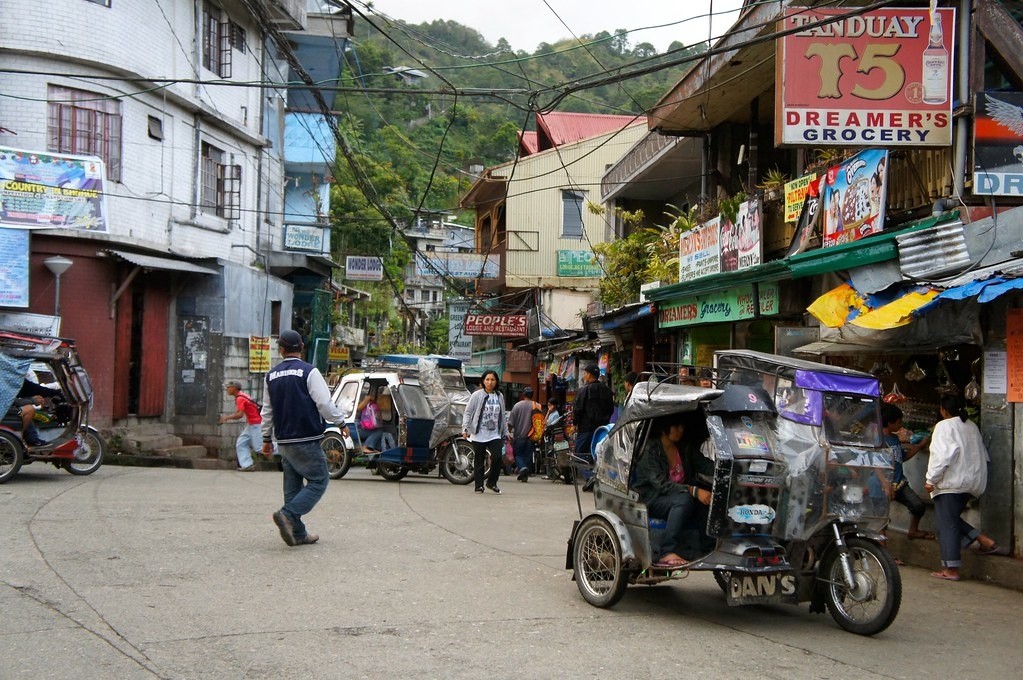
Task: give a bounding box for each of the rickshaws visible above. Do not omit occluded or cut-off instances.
[319,355,492,485]
[566,350,902,637]
[0,327,106,485]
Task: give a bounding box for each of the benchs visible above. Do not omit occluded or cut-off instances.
[593,484,698,530]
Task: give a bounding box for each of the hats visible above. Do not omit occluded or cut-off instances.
[585,365,600,376]
[523,387,533,394]
[226,381,242,389]
[622,372,640,382]
[276,330,302,348]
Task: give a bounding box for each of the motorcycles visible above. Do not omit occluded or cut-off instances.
[543,410,574,485]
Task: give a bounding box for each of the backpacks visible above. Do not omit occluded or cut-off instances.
[527,401,545,441]
[360,400,384,430]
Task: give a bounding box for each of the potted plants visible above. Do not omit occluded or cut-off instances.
[761,162,788,199]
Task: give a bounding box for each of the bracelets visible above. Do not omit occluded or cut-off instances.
[692,486,698,498]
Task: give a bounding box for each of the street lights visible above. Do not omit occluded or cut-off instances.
[43,256,73,317]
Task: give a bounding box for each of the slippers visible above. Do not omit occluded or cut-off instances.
[654,553,689,567]
[974,546,1000,555]
[908,530,935,540]
[930,571,961,581]
[894,559,906,565]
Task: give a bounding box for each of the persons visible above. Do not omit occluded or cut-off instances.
[355,394,383,453]
[869,403,936,565]
[507,386,543,482]
[219,379,279,472]
[462,370,512,494]
[632,412,717,566]
[572,365,615,493]
[541,398,560,480]
[14,378,57,447]
[736,207,759,250]
[624,371,641,391]
[827,188,843,234]
[260,329,350,546]
[925,393,1000,580]
[680,365,712,387]
[869,157,885,219]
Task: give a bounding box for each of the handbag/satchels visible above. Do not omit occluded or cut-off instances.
[869,361,981,405]
[503,441,514,465]
[258,405,262,413]
[895,427,930,444]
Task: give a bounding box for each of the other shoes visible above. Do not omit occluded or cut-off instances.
[475,486,484,493]
[28,438,53,448]
[582,476,595,491]
[541,475,549,480]
[517,469,528,483]
[273,510,297,546]
[486,483,501,494]
[296,531,319,544]
[237,464,255,472]
[362,445,380,453]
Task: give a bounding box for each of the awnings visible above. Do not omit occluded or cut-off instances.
[101,248,220,306]
